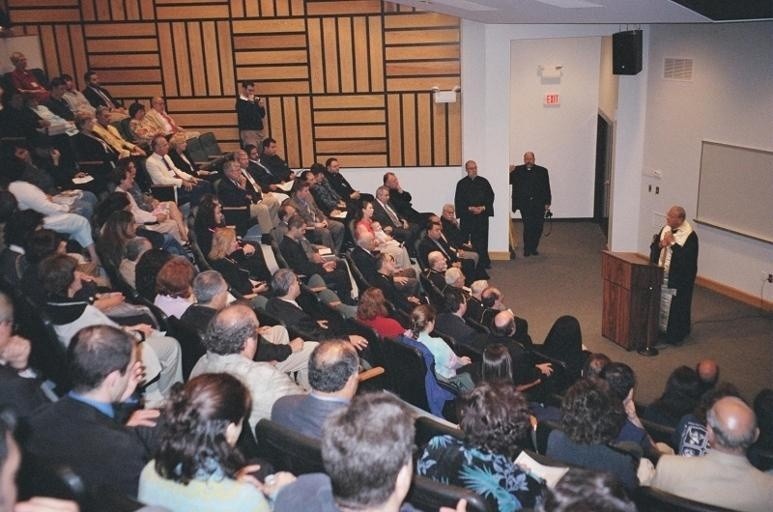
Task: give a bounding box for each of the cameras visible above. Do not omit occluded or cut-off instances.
[254,96,260,101]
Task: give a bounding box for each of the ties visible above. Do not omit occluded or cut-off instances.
[161,156,183,180]
[98,88,115,108]
[160,112,178,130]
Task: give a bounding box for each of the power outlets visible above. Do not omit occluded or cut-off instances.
[768,274,773,283]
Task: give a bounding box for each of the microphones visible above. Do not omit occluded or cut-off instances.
[650,234,659,248]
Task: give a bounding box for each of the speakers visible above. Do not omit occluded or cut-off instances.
[612,30,642,75]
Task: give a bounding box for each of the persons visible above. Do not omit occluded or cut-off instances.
[0,50,772,511]
